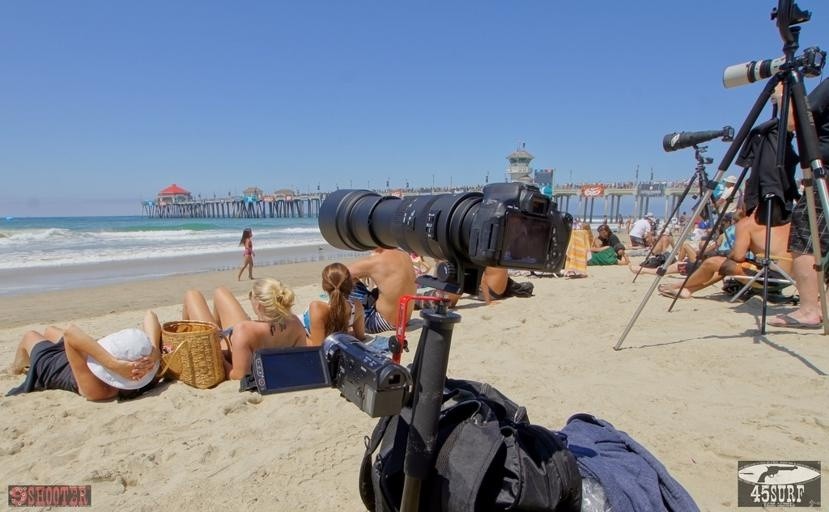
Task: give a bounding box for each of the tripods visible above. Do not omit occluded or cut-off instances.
[632,164,732,284]
[613,69,829,351]
[666,162,796,335]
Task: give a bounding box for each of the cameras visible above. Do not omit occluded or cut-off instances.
[239,331,413,418]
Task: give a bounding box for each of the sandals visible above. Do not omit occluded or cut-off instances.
[565,270,588,278]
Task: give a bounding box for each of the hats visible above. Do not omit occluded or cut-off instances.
[86,328,159,391]
[725,176,738,185]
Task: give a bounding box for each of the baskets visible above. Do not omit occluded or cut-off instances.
[157,320,234,389]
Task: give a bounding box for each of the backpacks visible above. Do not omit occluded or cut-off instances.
[359,372,587,511]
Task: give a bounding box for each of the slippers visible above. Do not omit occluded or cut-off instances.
[766,314,821,330]
[658,283,674,291]
[662,289,681,300]
